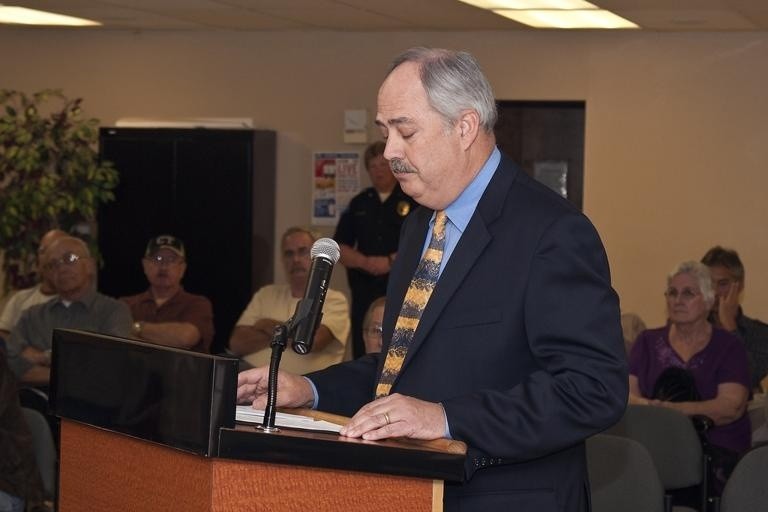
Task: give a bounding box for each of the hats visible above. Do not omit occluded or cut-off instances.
[144,233,185,258]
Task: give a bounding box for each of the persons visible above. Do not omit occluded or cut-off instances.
[362,294,386,354]
[700,245,768,404]
[236,46,631,511]
[228,225,351,376]
[334,140,419,360]
[1,338,46,512]
[626,259,752,511]
[8,234,132,387]
[0,228,71,342]
[117,235,214,350]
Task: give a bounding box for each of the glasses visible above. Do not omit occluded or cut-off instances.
[149,254,176,264]
[363,323,383,339]
[42,252,87,275]
[36,246,46,255]
[664,287,696,302]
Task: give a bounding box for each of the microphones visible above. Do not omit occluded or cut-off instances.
[292,237,344,355]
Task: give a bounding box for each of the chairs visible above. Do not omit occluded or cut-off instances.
[585,433,673,512]
[605,404,708,512]
[714,447,768,512]
[21,406,59,512]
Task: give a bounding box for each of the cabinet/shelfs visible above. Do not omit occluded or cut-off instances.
[97,126,277,353]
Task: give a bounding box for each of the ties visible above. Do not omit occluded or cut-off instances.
[375,211,447,398]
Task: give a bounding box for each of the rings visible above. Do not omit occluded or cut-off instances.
[383,412,391,425]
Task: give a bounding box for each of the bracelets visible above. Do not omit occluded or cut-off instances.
[43,345,53,366]
[133,319,143,338]
